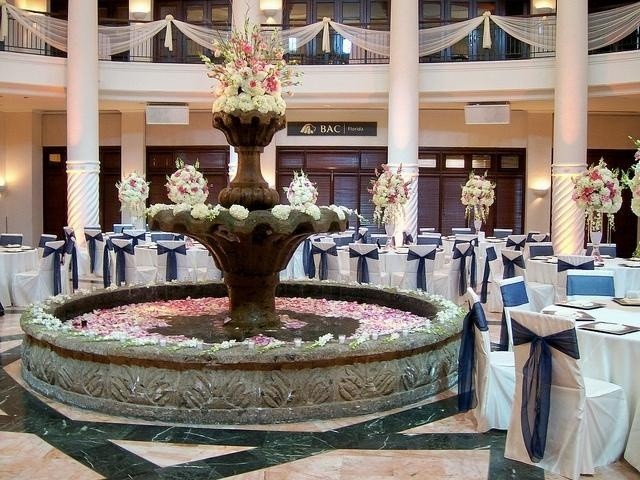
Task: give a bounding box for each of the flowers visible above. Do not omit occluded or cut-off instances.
[622,136,640,221]
[284,173,318,210]
[368,162,412,224]
[147,203,355,223]
[462,171,498,222]
[200,1,301,114]
[570,156,622,222]
[117,174,152,214]
[168,159,209,207]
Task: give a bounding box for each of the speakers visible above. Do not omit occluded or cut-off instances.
[145,106,189,124]
[464,104,510,124]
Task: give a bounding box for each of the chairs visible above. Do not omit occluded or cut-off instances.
[0,223,639,479]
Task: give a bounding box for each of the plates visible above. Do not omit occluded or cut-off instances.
[598,255,614,259]
[577,321,640,337]
[555,300,607,310]
[531,255,560,264]
[613,297,639,306]
[5,244,36,253]
[595,262,604,267]
[618,257,640,268]
[542,310,595,322]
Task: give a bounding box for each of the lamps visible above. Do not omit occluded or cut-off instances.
[133,12,147,19]
[263,8,277,19]
[536,8,554,14]
